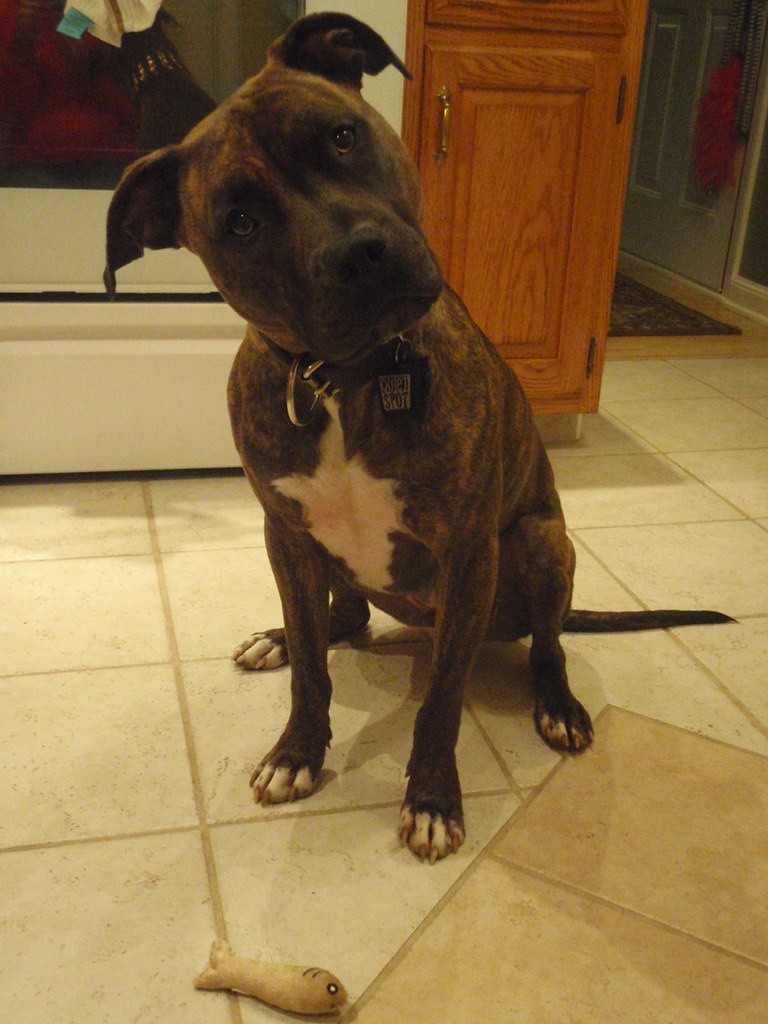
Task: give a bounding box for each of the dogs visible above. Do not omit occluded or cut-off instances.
[103,10,740,863]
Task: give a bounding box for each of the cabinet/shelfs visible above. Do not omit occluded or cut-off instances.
[403,1,646,444]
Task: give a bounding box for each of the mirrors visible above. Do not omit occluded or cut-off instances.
[0,0,306,194]
